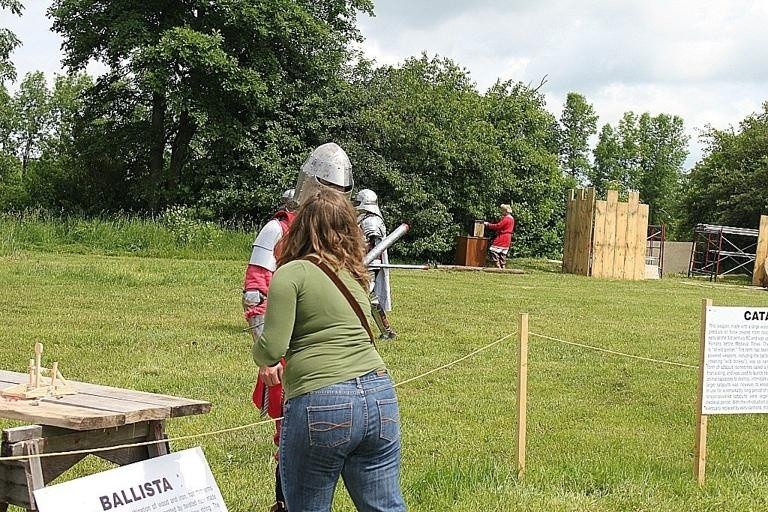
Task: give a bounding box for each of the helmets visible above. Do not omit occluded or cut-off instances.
[284,142,354,206]
[354,189,385,221]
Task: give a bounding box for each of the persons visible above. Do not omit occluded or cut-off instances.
[484,204,514,269]
[350,187,397,342]
[243,142,354,512]
[252,189,407,512]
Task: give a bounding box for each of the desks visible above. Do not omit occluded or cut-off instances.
[456,234,492,266]
[0,365,213,510]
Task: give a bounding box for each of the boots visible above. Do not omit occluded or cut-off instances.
[372,298,400,342]
